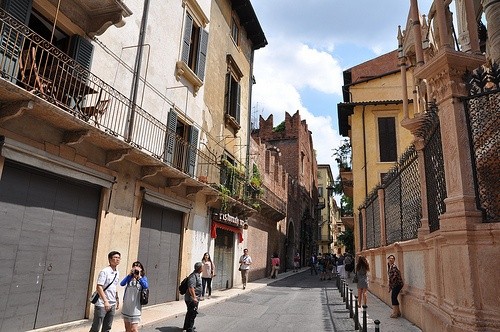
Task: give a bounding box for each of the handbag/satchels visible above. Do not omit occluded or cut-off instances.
[239,268,241,271]
[90,291,99,304]
[141,289,149,305]
[275,266,280,269]
[352,271,357,283]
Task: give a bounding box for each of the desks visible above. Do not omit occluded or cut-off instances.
[39,63,99,114]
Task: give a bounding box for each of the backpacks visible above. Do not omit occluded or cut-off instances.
[179,277,188,295]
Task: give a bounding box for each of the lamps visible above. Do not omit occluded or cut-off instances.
[243,224,248,230]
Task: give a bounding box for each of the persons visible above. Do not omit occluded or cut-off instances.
[270,252,280,279]
[356,256,370,308]
[239,249,252,289]
[387,255,403,318]
[309,252,339,281]
[120,261,148,332]
[183,261,204,332]
[200,252,215,300]
[90,251,121,332]
[293,253,300,272]
[338,252,354,278]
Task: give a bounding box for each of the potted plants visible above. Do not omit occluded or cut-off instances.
[338,163,351,172]
[250,176,261,190]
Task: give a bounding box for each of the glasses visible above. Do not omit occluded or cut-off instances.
[135,263,140,266]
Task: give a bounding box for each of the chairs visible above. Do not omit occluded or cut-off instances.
[81,99,111,129]
[18,47,57,106]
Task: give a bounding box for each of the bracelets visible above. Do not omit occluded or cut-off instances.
[116,297,118,299]
